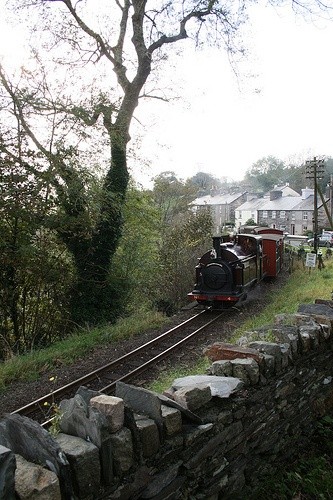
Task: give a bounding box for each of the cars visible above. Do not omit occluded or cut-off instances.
[306,234,333,247]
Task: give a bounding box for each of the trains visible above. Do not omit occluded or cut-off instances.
[187,224,284,315]
